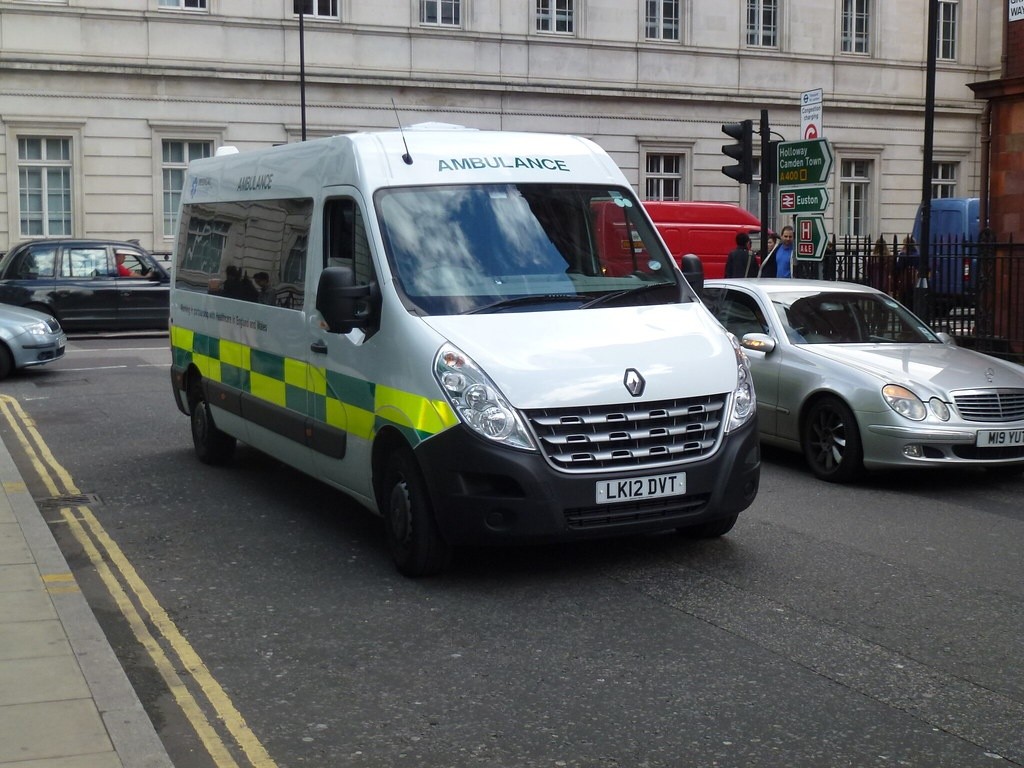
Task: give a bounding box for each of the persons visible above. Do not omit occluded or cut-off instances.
[866,237,920,331]
[116,254,152,278]
[768,226,835,281]
[723,233,760,279]
[208,266,282,308]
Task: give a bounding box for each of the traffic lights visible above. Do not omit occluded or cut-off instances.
[720,117,754,183]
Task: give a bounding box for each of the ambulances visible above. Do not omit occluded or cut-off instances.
[168,121,765,581]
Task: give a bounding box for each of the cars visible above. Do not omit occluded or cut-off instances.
[0,236,181,331]
[696,277,1024,486]
[0,300,68,382]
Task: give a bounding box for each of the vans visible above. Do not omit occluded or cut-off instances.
[899,197,989,315]
[583,200,785,284]
